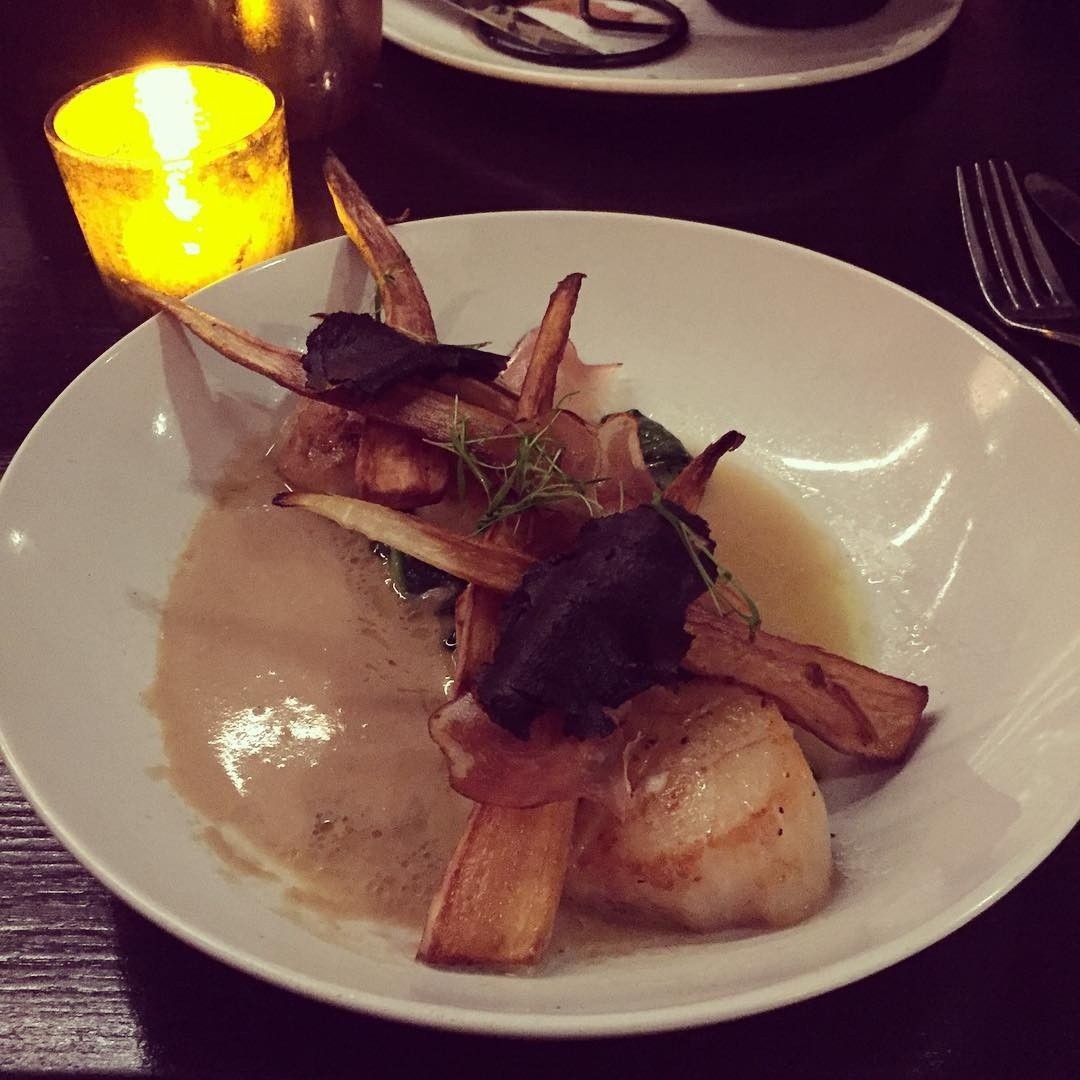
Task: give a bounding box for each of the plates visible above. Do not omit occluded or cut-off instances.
[337,0,963,94]
[1,208,1079,1038]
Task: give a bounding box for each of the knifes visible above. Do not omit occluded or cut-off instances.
[1024,171,1079,245]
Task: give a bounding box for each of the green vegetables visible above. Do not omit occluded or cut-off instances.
[420,394,762,633]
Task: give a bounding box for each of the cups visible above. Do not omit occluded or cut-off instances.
[44,60,294,322]
[152,1,384,145]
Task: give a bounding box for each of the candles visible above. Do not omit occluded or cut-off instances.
[54,58,289,305]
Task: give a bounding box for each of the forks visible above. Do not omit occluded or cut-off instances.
[957,161,1080,355]
